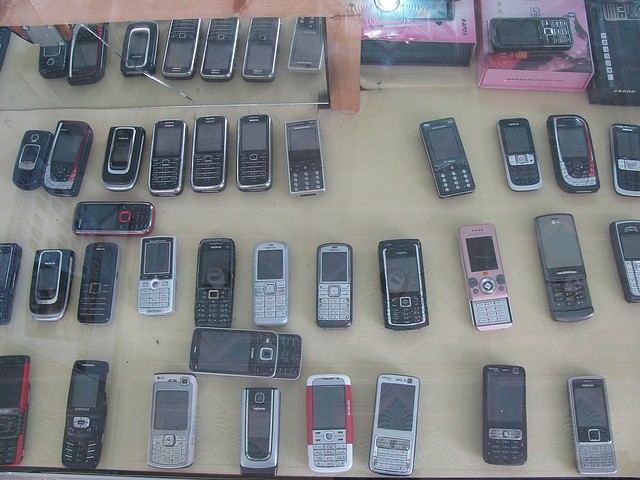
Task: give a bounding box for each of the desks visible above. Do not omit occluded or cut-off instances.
[0,0,368,114]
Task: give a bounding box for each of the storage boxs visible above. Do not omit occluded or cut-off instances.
[582,2,639,106]
[476,2,596,93]
[358,0,478,69]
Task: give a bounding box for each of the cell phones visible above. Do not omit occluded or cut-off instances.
[609,122,640,199]
[148,119,188,197]
[566,374,619,476]
[497,118,544,192]
[200,16,240,82]
[188,328,302,379]
[367,373,421,474]
[137,236,176,317]
[420,117,478,197]
[43,118,93,197]
[287,17,325,75]
[67,24,108,87]
[608,217,640,303]
[457,225,514,330]
[77,242,121,325]
[378,238,431,331]
[235,114,274,192]
[120,23,159,79]
[239,384,280,477]
[194,236,235,329]
[0,241,23,326]
[147,371,197,475]
[243,17,281,82]
[37,30,70,79]
[11,128,50,193]
[534,214,596,323]
[0,351,31,469]
[252,241,288,328]
[192,116,228,193]
[284,116,326,199]
[305,373,354,475]
[59,358,110,469]
[481,361,529,468]
[546,112,600,193]
[488,15,573,51]
[71,204,154,237]
[315,243,354,329]
[102,126,145,191]
[29,246,75,320]
[160,18,202,82]
